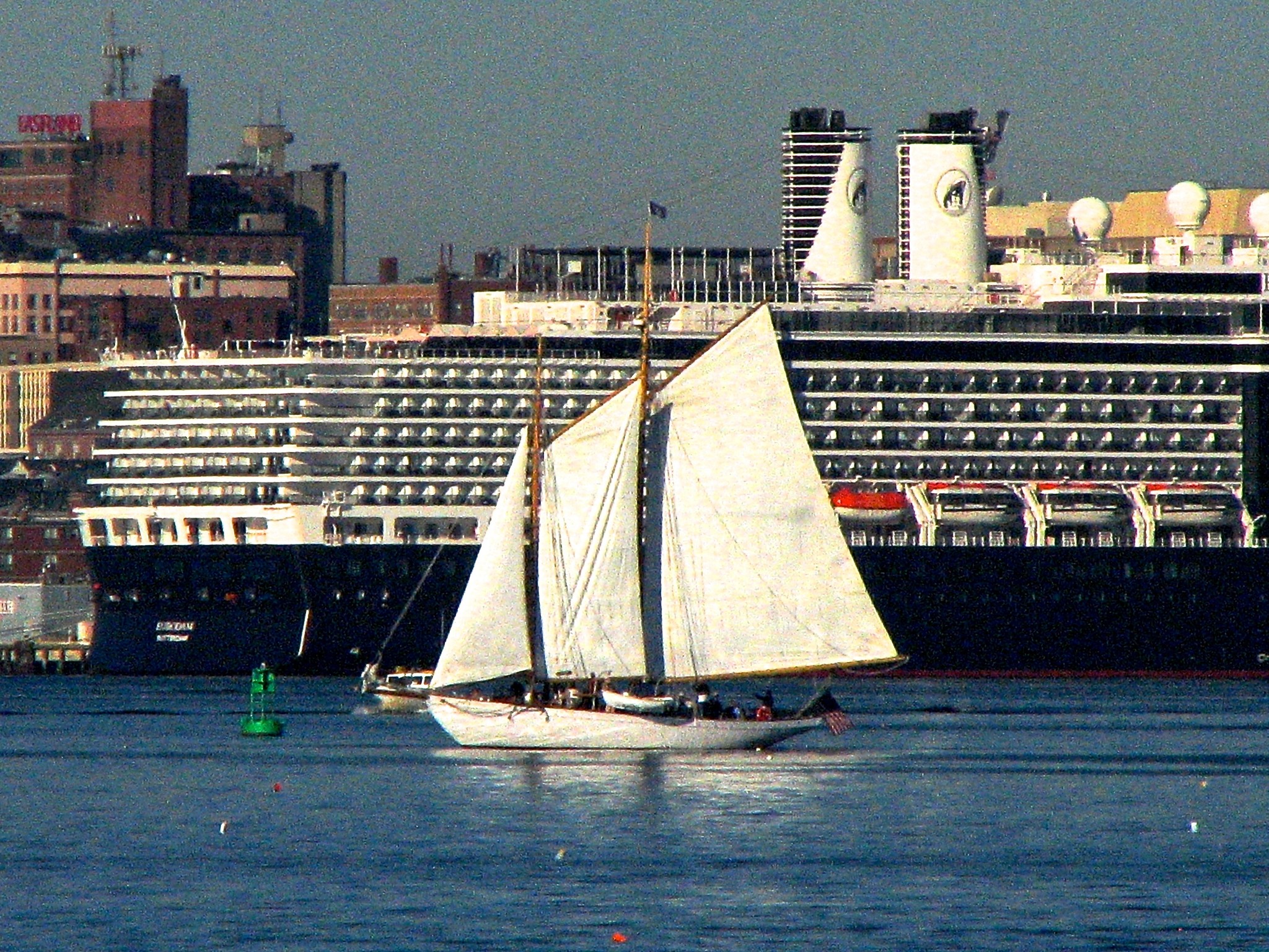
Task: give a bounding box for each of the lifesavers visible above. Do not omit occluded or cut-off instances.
[756,706,772,721]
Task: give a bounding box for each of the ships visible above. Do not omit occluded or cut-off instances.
[76,296,1268,681]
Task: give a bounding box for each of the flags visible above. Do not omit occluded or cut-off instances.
[649,201,667,219]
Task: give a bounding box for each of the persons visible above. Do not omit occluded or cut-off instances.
[754,689,773,710]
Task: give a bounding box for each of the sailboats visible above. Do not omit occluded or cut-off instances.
[422,206,905,750]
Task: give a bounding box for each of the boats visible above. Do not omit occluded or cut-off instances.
[1035,478,1129,527]
[834,487,908,524]
[923,483,1024,529]
[369,671,435,712]
[1143,483,1243,530]
